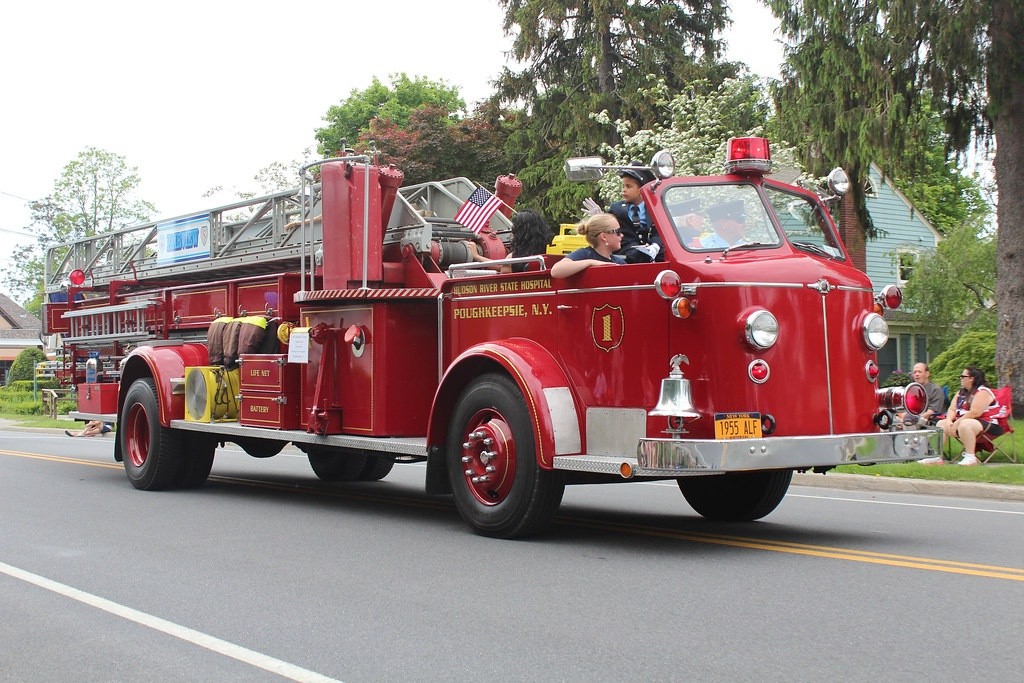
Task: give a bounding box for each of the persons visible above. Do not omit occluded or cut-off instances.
[467,161,751,279]
[65,420,114,437]
[897,363,1002,466]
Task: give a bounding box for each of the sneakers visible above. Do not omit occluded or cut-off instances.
[917,455,943,465]
[957,452,977,465]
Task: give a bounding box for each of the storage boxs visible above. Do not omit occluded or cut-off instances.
[77,383,119,414]
[234,352,302,430]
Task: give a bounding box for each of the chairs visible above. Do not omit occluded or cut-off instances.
[927,385,951,462]
[949,385,1017,463]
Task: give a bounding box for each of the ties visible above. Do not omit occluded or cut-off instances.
[631,206,641,229]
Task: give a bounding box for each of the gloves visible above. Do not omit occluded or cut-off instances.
[580,196,602,215]
[647,242,661,260]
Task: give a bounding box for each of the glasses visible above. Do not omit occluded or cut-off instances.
[959,375,970,379]
[594,227,622,237]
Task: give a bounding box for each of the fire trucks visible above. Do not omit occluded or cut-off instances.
[33,138,945,543]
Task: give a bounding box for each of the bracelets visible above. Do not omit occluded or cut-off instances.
[955,421,959,425]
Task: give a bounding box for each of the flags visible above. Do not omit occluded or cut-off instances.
[454,186,503,235]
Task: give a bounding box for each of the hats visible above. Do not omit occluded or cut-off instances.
[616,160,656,186]
[668,197,705,217]
[706,199,748,221]
